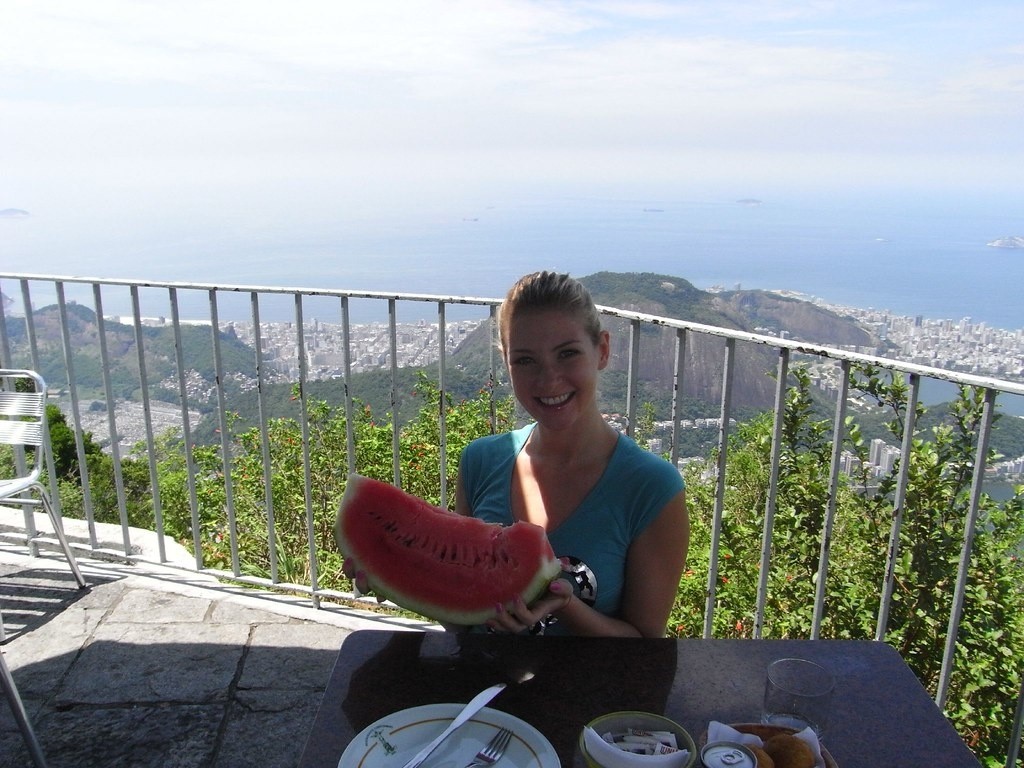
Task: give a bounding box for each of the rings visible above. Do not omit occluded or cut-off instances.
[528,614,560,636]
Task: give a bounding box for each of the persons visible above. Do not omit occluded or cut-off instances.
[342,270,691,639]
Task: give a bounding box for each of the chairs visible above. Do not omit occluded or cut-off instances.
[0,367,87,587]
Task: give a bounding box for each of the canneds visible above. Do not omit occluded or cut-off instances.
[697,740,758,768]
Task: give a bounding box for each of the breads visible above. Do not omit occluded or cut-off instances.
[742,743,775,768]
[763,733,817,768]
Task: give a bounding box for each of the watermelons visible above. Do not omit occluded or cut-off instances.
[330,472,561,626]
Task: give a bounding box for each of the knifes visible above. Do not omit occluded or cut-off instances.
[403,683,506,768]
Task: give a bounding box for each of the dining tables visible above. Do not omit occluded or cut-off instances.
[297,629,985,767]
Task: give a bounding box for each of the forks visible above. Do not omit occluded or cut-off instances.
[464,727,514,768]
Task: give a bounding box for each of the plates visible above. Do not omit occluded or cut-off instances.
[337,703,562,768]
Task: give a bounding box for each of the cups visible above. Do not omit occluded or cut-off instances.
[760,658,834,743]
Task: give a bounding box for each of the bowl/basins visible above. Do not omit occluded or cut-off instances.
[579,711,696,768]
[699,723,837,768]
